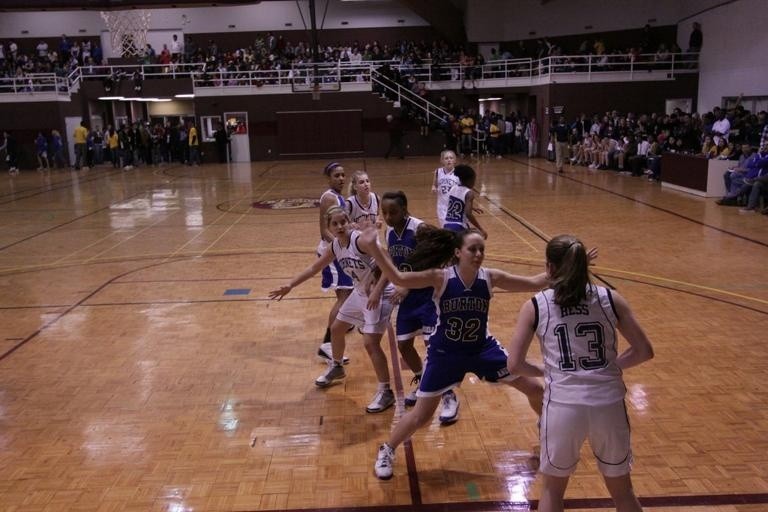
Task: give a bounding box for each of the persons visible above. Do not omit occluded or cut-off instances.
[507,235,654,512]
[432,151,458,228]
[356,214,598,478]
[381,190,461,423]
[345,170,386,231]
[1,22,768,215]
[444,165,489,241]
[317,161,354,366]
[267,205,396,413]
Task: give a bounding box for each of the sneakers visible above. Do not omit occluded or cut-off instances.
[366,389,395,413]
[316,342,350,363]
[374,445,394,479]
[439,394,461,422]
[315,364,348,387]
[405,374,422,406]
